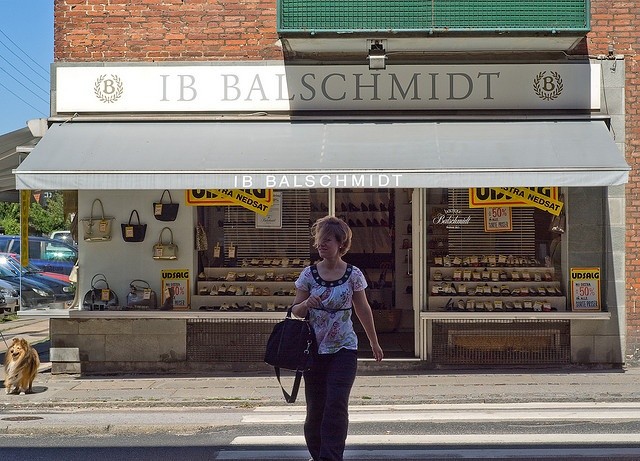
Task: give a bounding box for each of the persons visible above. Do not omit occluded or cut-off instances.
[289,216,384,461]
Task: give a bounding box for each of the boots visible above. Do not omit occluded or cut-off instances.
[226,245,237,267]
[211,246,224,267]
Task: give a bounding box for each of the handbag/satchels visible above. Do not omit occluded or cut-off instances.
[82,274,119,310]
[69,258,79,283]
[549,211,565,235]
[263,303,318,403]
[153,190,179,221]
[79,199,116,242]
[152,227,178,261]
[121,209,147,242]
[127,280,157,311]
[372,300,402,334]
[70,212,78,245]
[195,221,208,251]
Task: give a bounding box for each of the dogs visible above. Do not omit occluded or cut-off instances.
[3,337,40,395]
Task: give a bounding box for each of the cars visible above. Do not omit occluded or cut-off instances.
[51,231,78,247]
[0,254,76,301]
[0,252,73,283]
[0,280,20,315]
[0,254,56,308]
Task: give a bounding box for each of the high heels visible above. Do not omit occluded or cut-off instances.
[200,284,296,296]
[436,298,557,312]
[338,216,387,226]
[434,255,540,267]
[198,272,299,282]
[335,202,386,211]
[431,282,563,296]
[433,268,552,281]
[241,258,311,268]
[199,302,293,312]
[311,203,328,212]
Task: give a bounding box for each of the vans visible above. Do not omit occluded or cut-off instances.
[0,236,78,262]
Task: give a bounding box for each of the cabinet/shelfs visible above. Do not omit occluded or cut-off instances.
[190,260,309,312]
[399,201,447,295]
[427,255,568,318]
[351,263,401,334]
[202,190,393,259]
